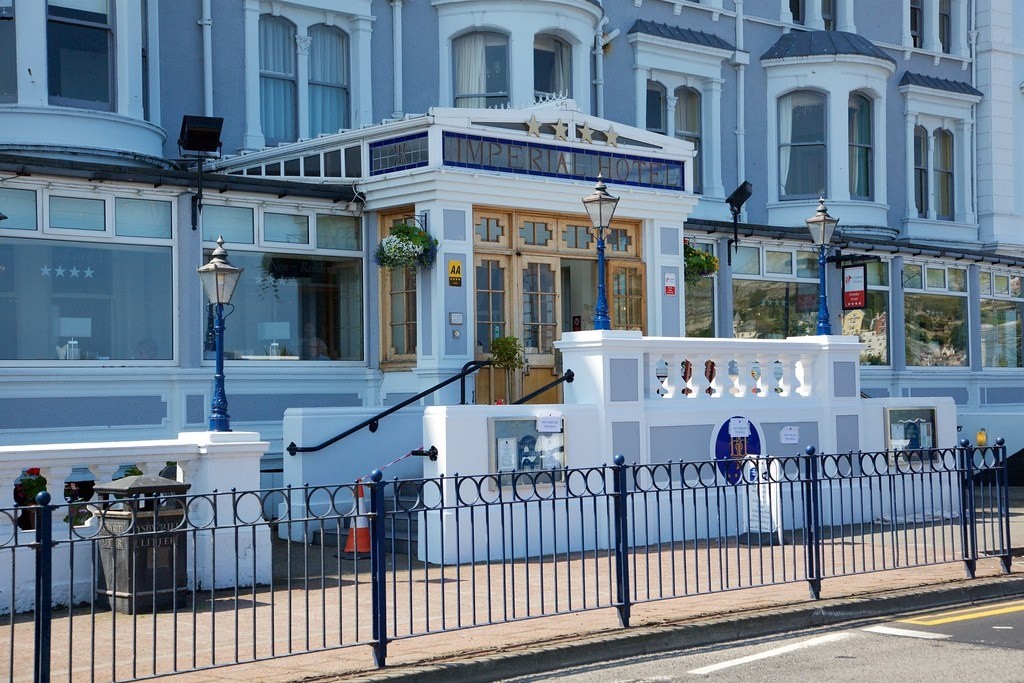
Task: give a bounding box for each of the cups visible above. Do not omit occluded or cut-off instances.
[67,341,79,359]
[270,342,280,361]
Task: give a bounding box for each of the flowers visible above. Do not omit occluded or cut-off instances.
[684,237,720,292]
[370,223,439,286]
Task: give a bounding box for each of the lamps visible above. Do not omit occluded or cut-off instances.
[177,116,225,232]
[725,181,753,267]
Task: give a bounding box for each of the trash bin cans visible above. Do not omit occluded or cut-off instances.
[93,474,193,615]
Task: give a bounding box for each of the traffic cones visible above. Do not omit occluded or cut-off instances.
[333,477,374,560]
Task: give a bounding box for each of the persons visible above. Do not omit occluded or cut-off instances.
[137,339,158,360]
[297,321,331,360]
